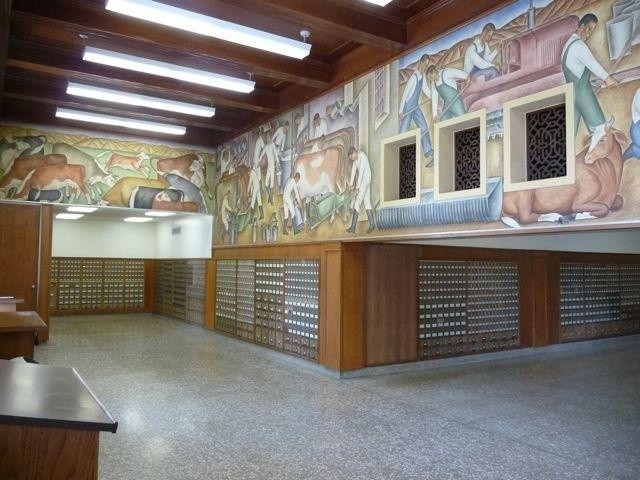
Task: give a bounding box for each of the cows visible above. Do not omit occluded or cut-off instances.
[1,132,215,215]
[288,124,357,229]
[498,112,628,228]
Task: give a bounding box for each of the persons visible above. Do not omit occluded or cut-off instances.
[560,13,621,137]
[218,113,377,243]
[622,86,640,164]
[397,23,500,167]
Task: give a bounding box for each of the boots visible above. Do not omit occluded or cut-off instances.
[365,209,375,233]
[346,209,359,233]
[283,216,301,236]
[247,204,264,224]
[424,150,434,167]
[265,185,276,206]
[221,230,228,243]
